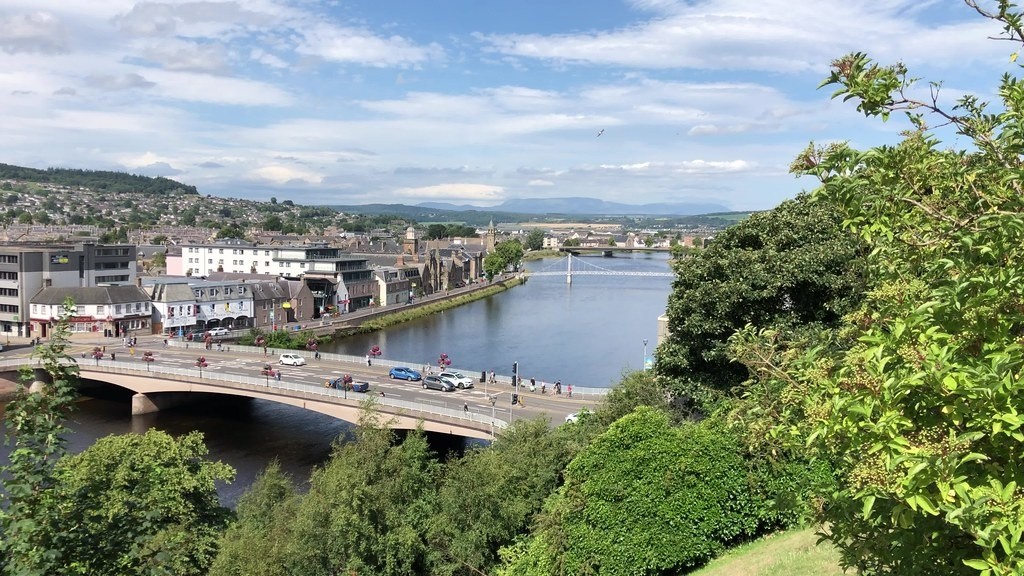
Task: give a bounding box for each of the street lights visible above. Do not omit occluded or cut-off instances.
[643,339,649,373]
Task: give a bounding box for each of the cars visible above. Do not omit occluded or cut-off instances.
[389,366,422,382]
[279,353,306,367]
[207,327,231,336]
[565,411,596,424]
[439,371,475,390]
[422,375,456,392]
[184,328,210,342]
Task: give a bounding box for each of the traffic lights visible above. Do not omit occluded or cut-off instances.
[512,362,517,373]
[512,394,518,405]
[511,375,517,386]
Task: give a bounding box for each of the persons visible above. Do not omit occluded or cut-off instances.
[122,334,136,347]
[277,371,281,380]
[488,370,495,385]
[542,381,545,393]
[216,341,222,351]
[568,384,572,397]
[464,402,468,411]
[163,339,168,348]
[264,346,267,354]
[315,349,318,359]
[552,380,561,395]
[531,377,535,391]
